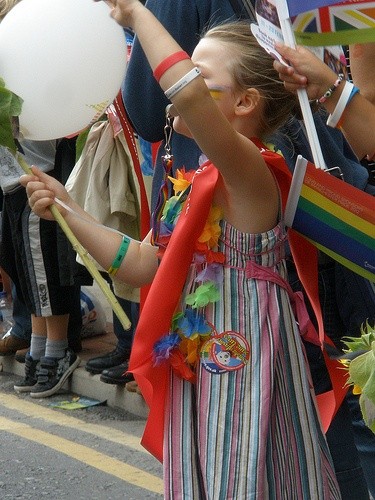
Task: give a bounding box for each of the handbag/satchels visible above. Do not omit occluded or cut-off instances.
[79,286,107,339]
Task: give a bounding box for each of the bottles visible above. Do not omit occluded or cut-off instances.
[0,292,15,336]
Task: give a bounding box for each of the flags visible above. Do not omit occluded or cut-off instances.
[285,0,375,46]
[283,155,375,283]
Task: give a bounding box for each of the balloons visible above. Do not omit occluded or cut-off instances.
[0,0,127,141]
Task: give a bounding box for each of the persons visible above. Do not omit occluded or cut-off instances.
[124,0,375,500]
[0,0,141,399]
[17,0,341,500]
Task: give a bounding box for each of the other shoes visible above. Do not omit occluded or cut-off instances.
[0,336,30,356]
[16,349,29,363]
[100,359,135,384]
[86,349,131,374]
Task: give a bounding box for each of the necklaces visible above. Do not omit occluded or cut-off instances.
[153,141,285,383]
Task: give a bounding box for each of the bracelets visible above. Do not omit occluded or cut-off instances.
[316,73,344,105]
[108,235,130,276]
[152,50,190,84]
[326,80,360,128]
[163,67,201,99]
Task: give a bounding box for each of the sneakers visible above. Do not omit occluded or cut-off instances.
[14,351,40,391]
[29,348,81,397]
